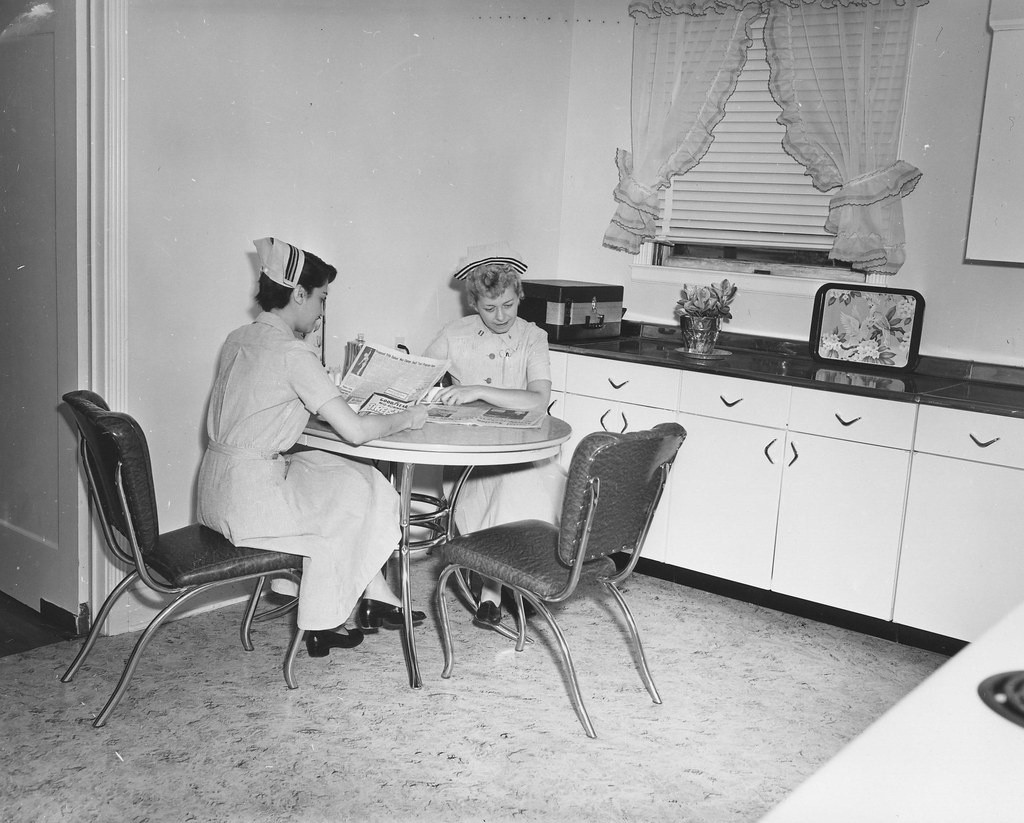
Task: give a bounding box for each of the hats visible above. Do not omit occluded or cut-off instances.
[453,241,528,281]
[253,237,305,289]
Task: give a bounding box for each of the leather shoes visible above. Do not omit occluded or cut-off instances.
[506,587,536,618]
[476,583,501,625]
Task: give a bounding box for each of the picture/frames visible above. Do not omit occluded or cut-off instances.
[808,282,925,376]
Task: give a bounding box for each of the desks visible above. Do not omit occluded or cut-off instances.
[295,400,573,691]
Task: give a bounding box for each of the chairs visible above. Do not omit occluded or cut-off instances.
[59,390,305,728]
[435,422,687,738]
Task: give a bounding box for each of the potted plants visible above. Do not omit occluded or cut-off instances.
[673,278,737,354]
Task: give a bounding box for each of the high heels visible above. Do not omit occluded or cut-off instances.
[307,629,365,658]
[360,595,427,629]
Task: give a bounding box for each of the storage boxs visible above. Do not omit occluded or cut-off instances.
[517,278,625,343]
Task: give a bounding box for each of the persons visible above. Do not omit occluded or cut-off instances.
[195,237,428,658]
[419,256,572,628]
[357,349,372,374]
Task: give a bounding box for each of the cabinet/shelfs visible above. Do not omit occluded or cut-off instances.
[541,351,1024,658]
